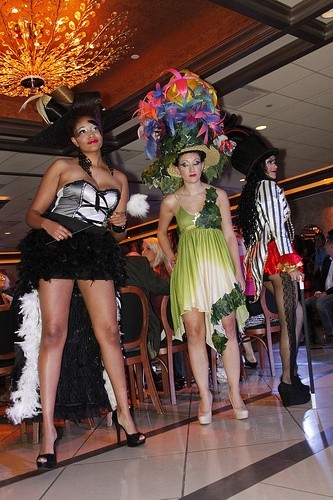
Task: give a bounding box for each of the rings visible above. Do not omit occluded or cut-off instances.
[58,235,62,238]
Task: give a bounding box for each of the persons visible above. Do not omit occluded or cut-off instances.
[239,154,310,405]
[121,237,194,396]
[295,229,333,341]
[235,232,279,367]
[10,114,147,468]
[0,275,13,304]
[157,148,249,424]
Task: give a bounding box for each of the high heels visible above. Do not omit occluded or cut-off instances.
[244,355,258,368]
[227,391,248,419]
[278,381,311,407]
[198,390,213,424]
[36,425,63,470]
[279,374,310,392]
[112,408,146,447]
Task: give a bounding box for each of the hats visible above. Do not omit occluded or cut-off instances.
[229,124,280,180]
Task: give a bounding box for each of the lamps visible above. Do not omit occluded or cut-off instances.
[0,0,138,96]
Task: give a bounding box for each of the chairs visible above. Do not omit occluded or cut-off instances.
[0,286,281,445]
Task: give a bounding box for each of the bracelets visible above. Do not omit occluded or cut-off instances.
[111,223,127,233]
